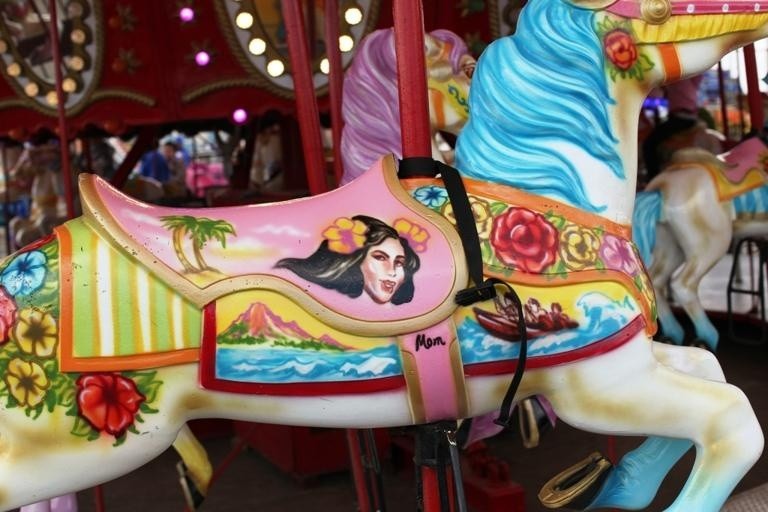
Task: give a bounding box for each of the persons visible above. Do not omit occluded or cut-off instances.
[660,71,705,136]
[140,136,192,202]
